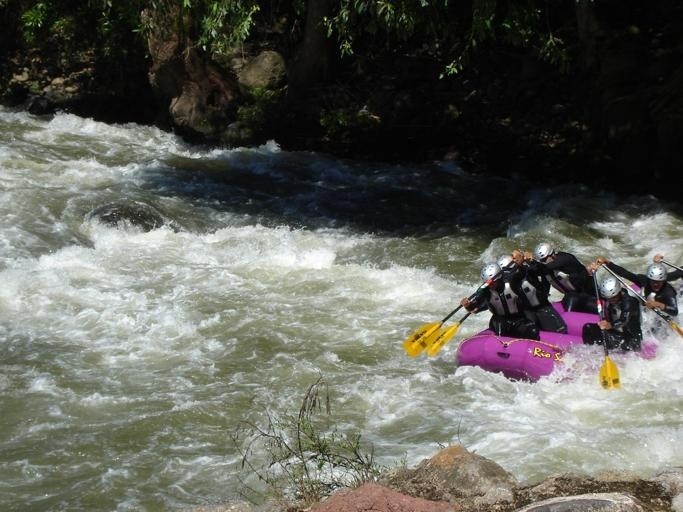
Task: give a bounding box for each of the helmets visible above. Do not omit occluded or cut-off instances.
[646,263,667,281]
[534,242,553,260]
[599,277,622,299]
[480,255,516,282]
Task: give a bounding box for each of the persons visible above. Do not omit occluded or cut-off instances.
[460,243,683,355]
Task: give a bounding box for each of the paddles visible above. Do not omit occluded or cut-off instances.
[591,267,620,392]
[426,291,487,359]
[598,258,683,339]
[401,254,514,358]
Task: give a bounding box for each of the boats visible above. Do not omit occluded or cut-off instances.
[456,283,680,383]
